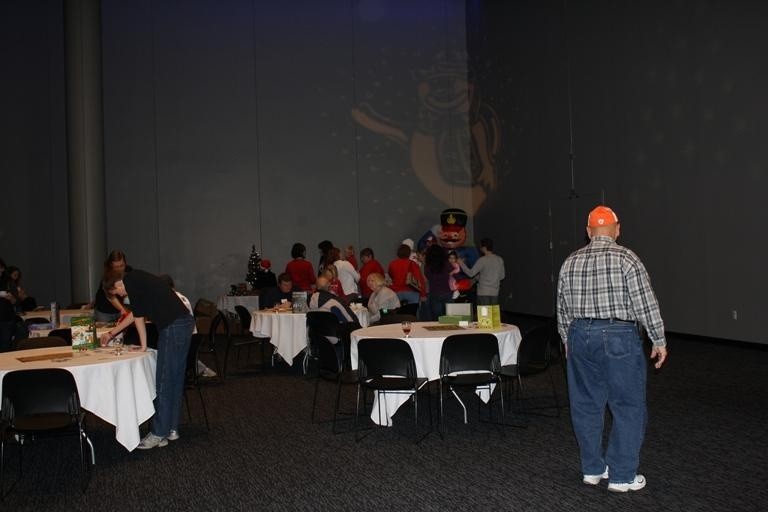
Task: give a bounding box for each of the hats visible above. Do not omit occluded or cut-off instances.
[588,205,618,228]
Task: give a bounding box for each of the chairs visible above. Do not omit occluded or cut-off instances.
[24,316,52,328]
[440,332,501,442]
[0,369,89,467]
[47,328,73,346]
[306,310,361,436]
[233,304,252,335]
[354,337,433,444]
[216,309,268,373]
[500,323,562,430]
[184,332,235,433]
[190,299,220,367]
[122,321,159,352]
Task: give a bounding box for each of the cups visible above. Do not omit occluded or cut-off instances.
[273,303,280,315]
[401,321,412,339]
[112,338,123,356]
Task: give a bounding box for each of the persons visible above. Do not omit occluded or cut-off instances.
[424,244,455,322]
[327,247,361,295]
[307,277,362,330]
[367,273,401,324]
[286,243,316,307]
[388,244,427,317]
[359,248,385,298]
[0,258,6,278]
[94,249,133,322]
[267,272,301,308]
[342,244,358,270]
[0,266,25,314]
[103,269,196,450]
[317,240,333,277]
[256,259,277,287]
[415,247,431,321]
[556,206,668,494]
[457,238,505,321]
[160,275,198,377]
[447,252,460,300]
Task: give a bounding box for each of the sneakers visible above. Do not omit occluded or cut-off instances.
[166,429,179,441]
[583,465,609,485]
[137,432,169,449]
[606,475,646,493]
